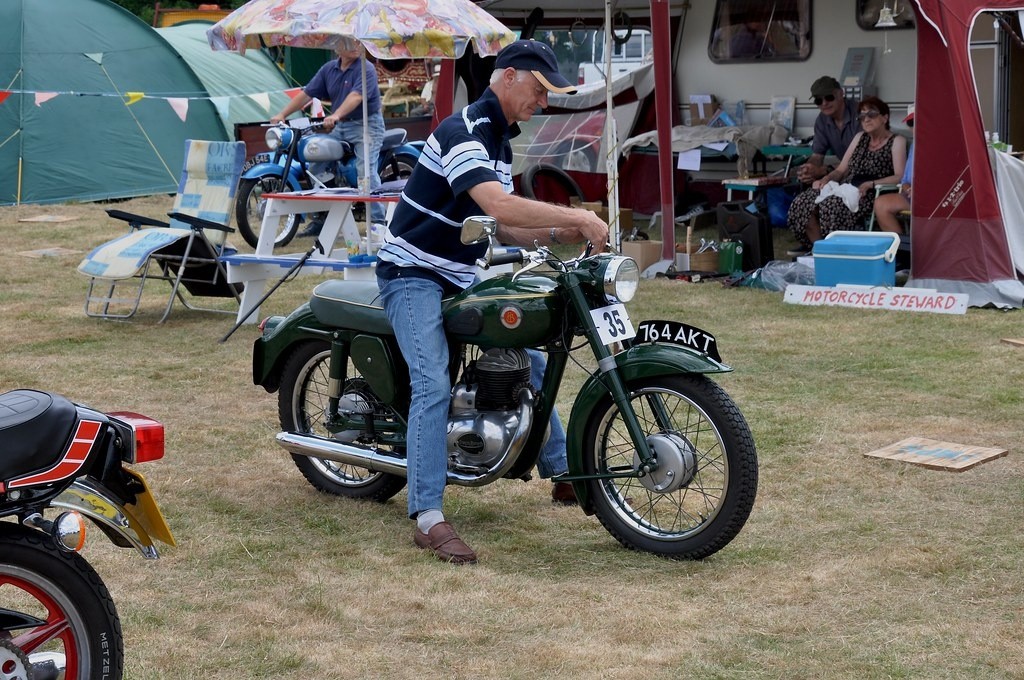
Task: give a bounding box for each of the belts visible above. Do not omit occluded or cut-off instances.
[339,108,381,122]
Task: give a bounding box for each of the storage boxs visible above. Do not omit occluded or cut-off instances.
[580,202,719,274]
[690,102,720,126]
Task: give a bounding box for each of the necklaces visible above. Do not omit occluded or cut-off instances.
[866,134,889,149]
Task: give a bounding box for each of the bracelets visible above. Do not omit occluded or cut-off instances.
[872,180,875,189]
[549,226,562,246]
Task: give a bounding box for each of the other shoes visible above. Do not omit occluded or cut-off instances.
[787,245,811,256]
[895,269,910,283]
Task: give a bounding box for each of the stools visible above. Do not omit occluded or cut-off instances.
[722,174,789,203]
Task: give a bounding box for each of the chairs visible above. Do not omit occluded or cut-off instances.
[867,184,910,248]
[77,139,248,326]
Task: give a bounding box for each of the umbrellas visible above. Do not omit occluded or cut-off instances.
[204,0,518,255]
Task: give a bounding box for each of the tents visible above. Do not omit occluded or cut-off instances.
[0,0,304,206]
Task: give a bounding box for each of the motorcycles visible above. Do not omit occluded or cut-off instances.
[251,215,760,562]
[0,387,176,680]
[234,121,427,250]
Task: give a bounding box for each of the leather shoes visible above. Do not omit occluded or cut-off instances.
[551,483,634,506]
[413,521,476,564]
[296,222,324,237]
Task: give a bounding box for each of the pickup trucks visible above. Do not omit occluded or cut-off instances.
[576,29,654,85]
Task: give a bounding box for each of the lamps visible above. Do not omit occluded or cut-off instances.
[875,0,897,27]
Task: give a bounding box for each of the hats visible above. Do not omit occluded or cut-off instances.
[495,38,578,95]
[902,104,914,124]
[432,64,441,77]
[809,76,839,101]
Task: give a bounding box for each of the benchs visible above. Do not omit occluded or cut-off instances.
[218,254,380,272]
[486,245,526,255]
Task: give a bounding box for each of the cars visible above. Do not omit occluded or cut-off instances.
[525,110,607,172]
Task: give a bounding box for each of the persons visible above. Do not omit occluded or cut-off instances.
[786,74,915,276]
[374,38,608,566]
[272,37,387,237]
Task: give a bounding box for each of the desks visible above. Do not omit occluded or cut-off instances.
[761,146,833,171]
[632,144,765,226]
[236,191,400,323]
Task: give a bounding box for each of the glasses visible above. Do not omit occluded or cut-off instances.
[814,93,836,106]
[906,119,914,127]
[856,110,880,121]
